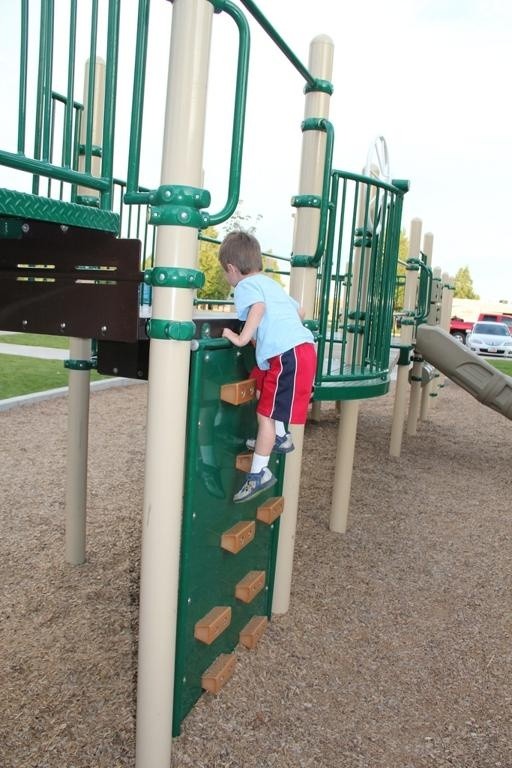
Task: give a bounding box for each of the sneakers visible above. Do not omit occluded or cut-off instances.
[233,466,277,504]
[245,431,293,454]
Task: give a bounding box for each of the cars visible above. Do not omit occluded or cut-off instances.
[465,321,512,357]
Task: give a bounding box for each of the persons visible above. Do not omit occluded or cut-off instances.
[217,229,318,504]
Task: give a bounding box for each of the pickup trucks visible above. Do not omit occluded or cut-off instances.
[451,311,512,345]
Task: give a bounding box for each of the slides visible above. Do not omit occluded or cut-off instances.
[416,323,511,420]
